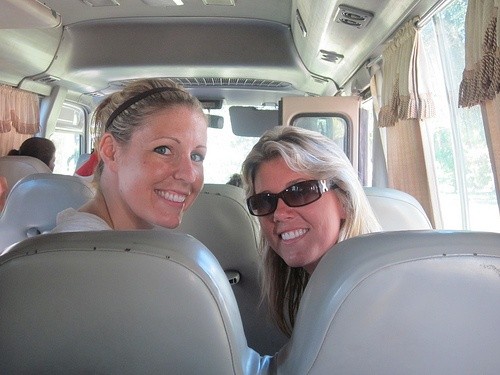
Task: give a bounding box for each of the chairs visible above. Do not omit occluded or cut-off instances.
[0,155,500,375]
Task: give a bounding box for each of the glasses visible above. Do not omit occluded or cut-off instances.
[247,179,339,217]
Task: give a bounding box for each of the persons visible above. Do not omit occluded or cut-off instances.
[241,125,382,341]
[7,137,56,172]
[0,80,207,255]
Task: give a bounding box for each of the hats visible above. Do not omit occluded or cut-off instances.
[75,151,97,177]
